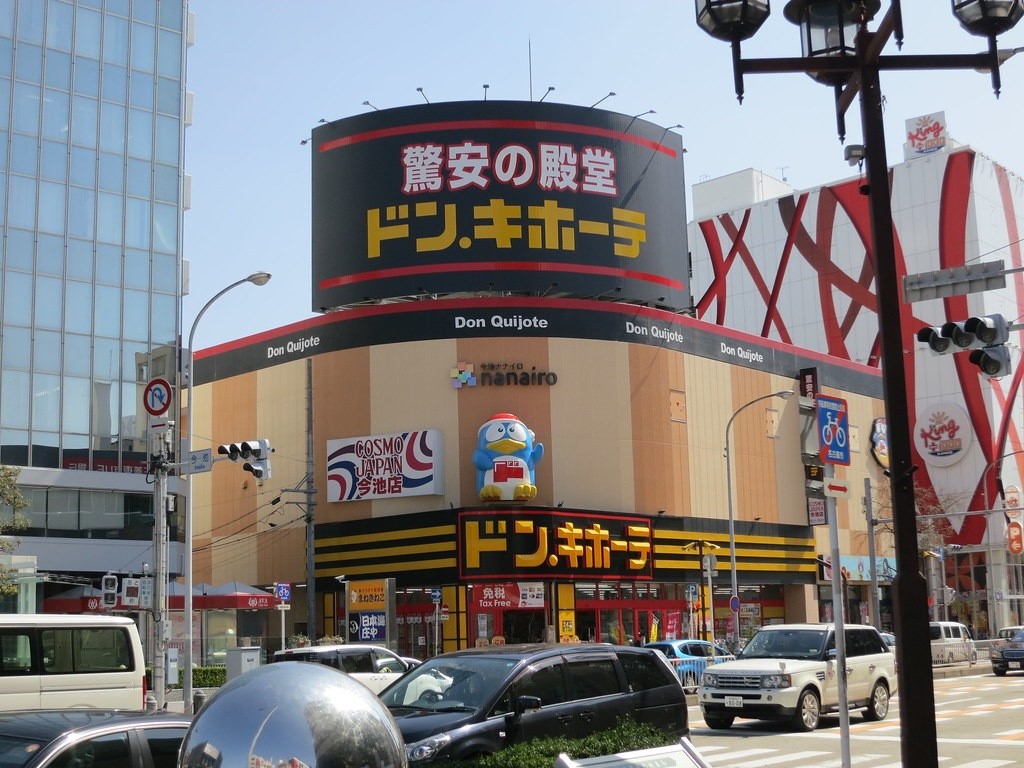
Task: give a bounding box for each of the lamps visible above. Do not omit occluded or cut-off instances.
[540,86,556,102]
[681,148,689,153]
[319,119,332,125]
[633,110,658,120]
[642,296,666,304]
[417,87,430,104]
[482,84,490,103]
[299,138,313,145]
[592,91,616,108]
[320,307,329,311]
[362,101,380,112]
[487,282,495,297]
[594,286,623,300]
[666,124,684,131]
[676,305,697,312]
[417,286,438,300]
[363,296,373,301]
[539,282,560,298]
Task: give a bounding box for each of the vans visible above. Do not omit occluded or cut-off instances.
[376,645,692,768]
[0,614,148,712]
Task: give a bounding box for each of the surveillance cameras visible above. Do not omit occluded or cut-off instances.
[335,575,345,580]
[704,542,720,550]
[682,542,698,552]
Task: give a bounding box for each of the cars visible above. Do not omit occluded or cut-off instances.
[373,657,454,695]
[643,640,736,694]
[929,621,977,664]
[274,645,444,704]
[990,628,1024,676]
[995,626,1024,649]
[0,708,194,768]
[880,633,898,672]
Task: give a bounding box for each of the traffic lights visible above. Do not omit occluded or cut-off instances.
[242,463,272,481]
[968,346,1012,378]
[946,544,964,552]
[218,439,270,462]
[917,313,1009,357]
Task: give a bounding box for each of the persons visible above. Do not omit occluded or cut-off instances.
[634,633,645,647]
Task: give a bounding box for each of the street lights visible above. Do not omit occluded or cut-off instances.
[183,273,273,717]
[698,0,1024,768]
[725,390,796,650]
[682,540,721,642]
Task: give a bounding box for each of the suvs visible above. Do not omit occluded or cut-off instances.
[696,622,899,731]
